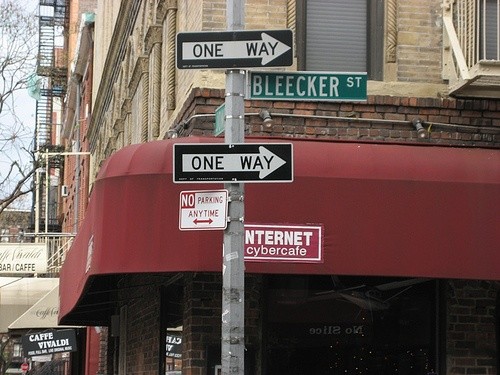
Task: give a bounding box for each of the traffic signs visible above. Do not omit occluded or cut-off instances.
[179,189,229,231]
[174,143,294,183]
[215,104,225,136]
[176,30,294,70]
[244,71,369,101]
